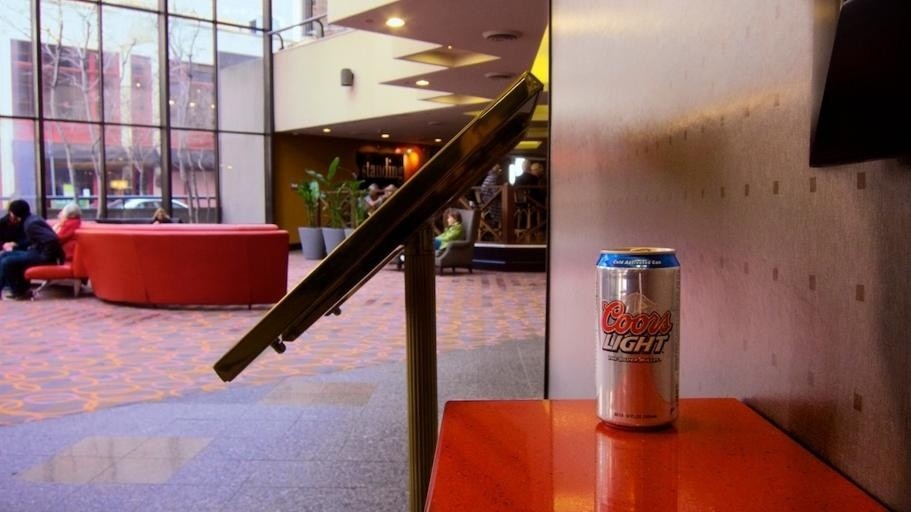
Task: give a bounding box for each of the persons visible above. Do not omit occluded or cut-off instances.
[150,208,172,224]
[365,184,397,217]
[0,200,65,300]
[481,164,503,228]
[435,212,464,251]
[514,163,545,208]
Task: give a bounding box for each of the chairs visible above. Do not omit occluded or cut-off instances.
[435,208,480,276]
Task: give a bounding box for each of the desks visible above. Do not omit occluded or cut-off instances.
[423,397,892,512]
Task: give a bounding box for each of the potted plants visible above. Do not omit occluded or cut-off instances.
[291,155,369,260]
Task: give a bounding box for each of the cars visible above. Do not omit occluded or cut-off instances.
[106,197,191,210]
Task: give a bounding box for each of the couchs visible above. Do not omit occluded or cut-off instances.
[82,221,290,305]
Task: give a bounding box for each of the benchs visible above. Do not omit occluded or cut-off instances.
[24,218,82,300]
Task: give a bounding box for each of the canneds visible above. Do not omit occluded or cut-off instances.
[594,246,682,428]
